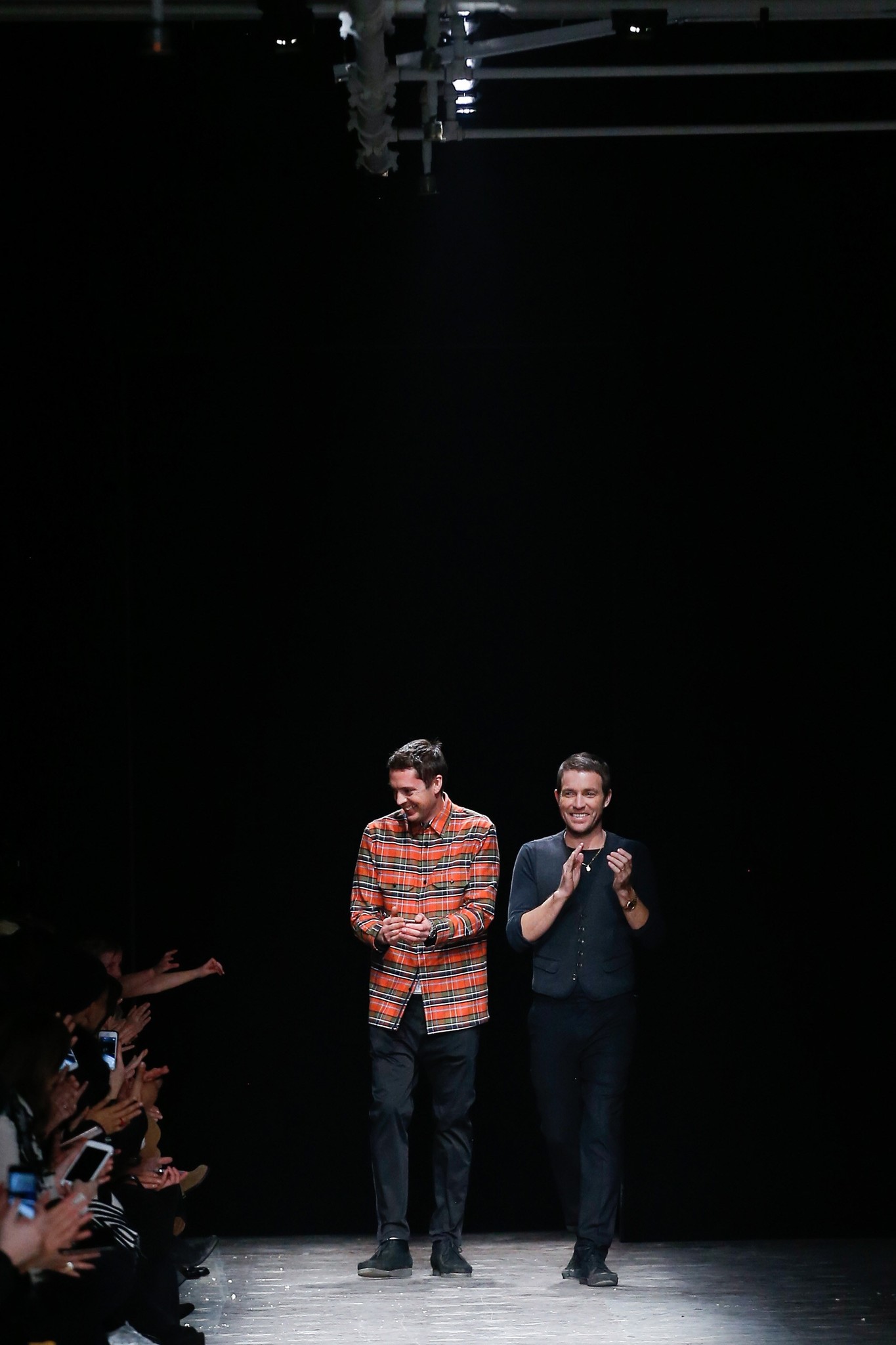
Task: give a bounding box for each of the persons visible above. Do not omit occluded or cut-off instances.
[349,739,501,1278]
[506,751,659,1286]
[0,935,224,1345]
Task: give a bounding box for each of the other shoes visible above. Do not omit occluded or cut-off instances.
[579,1246,618,1286]
[160,1324,205,1345]
[358,1239,413,1277]
[178,1302,195,1320]
[168,1234,219,1268]
[431,1236,472,1277]
[560,1250,582,1279]
[178,1265,210,1279]
[179,1164,208,1195]
[563,1222,578,1238]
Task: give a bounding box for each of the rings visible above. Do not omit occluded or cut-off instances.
[119,1118,126,1127]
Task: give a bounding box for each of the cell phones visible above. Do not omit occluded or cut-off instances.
[6,1163,42,1220]
[59,1049,80,1075]
[97,1030,119,1071]
[152,1168,185,1175]
[62,1125,103,1147]
[60,1140,115,1188]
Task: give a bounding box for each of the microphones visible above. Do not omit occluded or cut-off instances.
[425,842,428,847]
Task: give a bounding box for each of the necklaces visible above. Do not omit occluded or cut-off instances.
[564,831,603,871]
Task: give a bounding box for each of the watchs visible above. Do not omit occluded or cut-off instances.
[429,920,437,940]
[623,889,637,910]
